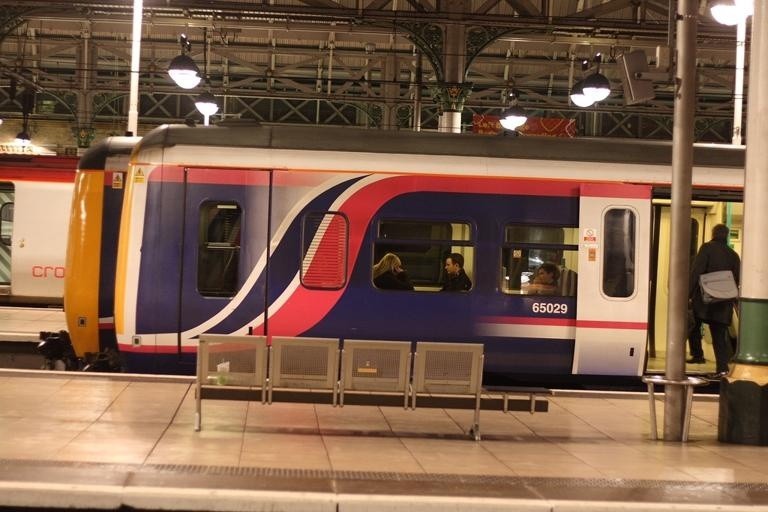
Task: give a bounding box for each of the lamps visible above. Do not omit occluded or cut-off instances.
[497,84,528,131]
[569,52,613,108]
[167,34,220,118]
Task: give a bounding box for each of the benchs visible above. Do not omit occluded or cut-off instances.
[192,332,487,443]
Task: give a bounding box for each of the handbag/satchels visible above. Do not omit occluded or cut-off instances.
[698,270,739,305]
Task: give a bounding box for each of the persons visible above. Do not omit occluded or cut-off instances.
[523,263,561,294]
[439,252,472,294]
[372,252,410,290]
[689,224,740,378]
[685,296,707,364]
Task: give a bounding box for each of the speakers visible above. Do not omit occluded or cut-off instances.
[615,49,655,107]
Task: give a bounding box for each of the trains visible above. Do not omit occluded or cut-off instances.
[0,121,767,391]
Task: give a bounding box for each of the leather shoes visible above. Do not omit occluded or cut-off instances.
[711,370,728,378]
[687,356,704,364]
